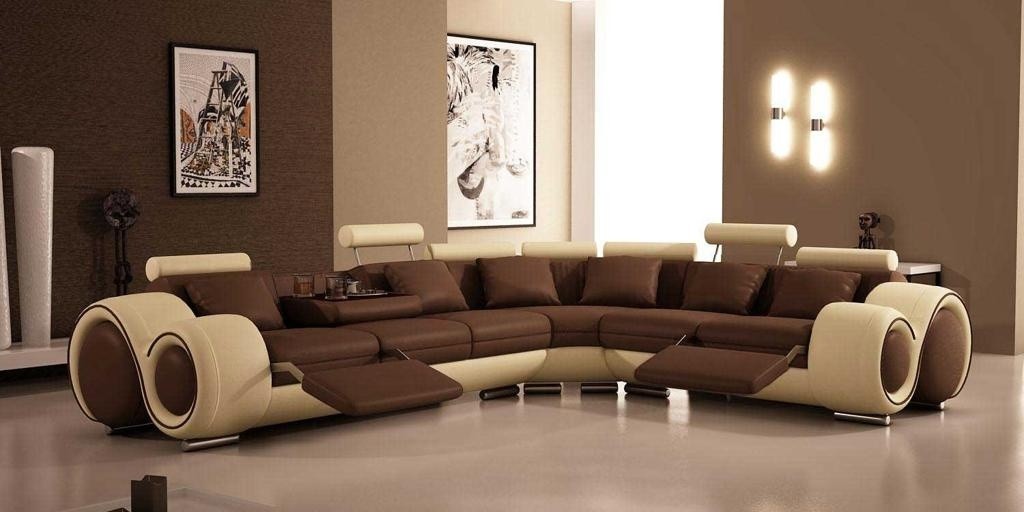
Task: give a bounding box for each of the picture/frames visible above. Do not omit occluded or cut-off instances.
[444,32,539,230]
[167,42,260,199]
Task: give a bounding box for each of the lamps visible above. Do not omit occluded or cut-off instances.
[809,78,839,174]
[771,67,795,161]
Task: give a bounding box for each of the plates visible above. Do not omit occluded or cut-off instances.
[346,290,388,297]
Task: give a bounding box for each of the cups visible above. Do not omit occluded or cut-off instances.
[292,273,315,297]
[323,277,348,300]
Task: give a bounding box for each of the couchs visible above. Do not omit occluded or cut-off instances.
[67,220,974,450]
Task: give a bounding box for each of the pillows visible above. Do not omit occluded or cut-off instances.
[338,223,424,246]
[797,245,899,274]
[476,256,563,307]
[705,223,799,250]
[145,252,254,283]
[424,242,516,261]
[579,256,662,310]
[281,292,423,326]
[768,269,860,318]
[682,261,768,314]
[523,240,600,260]
[184,274,286,332]
[604,240,696,261]
